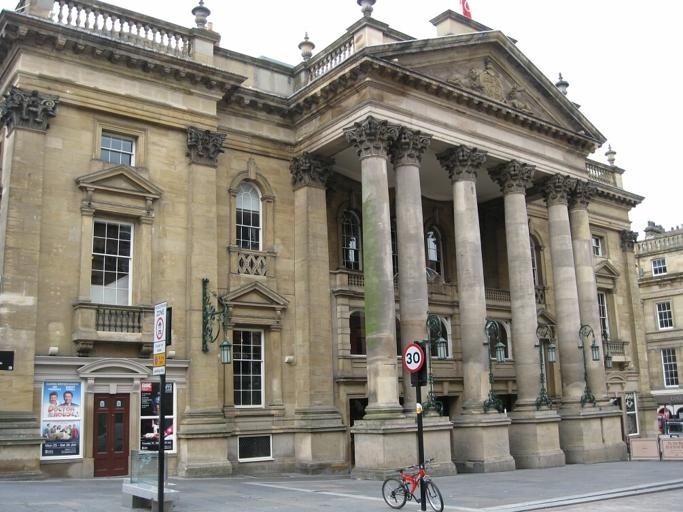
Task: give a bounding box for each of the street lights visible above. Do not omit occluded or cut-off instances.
[535,325,557,412]
[423,313,448,416]
[602,329,617,406]
[577,324,601,409]
[483,319,506,414]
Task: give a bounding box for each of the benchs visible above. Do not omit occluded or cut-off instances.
[122,483,180,512]
[123,478,176,507]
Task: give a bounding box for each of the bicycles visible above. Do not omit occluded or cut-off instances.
[382,457,445,512]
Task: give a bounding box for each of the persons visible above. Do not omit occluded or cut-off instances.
[45,391,60,416]
[43,422,78,442]
[53,390,79,408]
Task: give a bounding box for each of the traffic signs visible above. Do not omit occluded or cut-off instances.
[402,343,424,373]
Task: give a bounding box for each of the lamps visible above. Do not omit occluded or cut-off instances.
[603,330,613,369]
[202,278,232,364]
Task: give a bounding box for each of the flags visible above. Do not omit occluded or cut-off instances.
[461,0,472,18]
